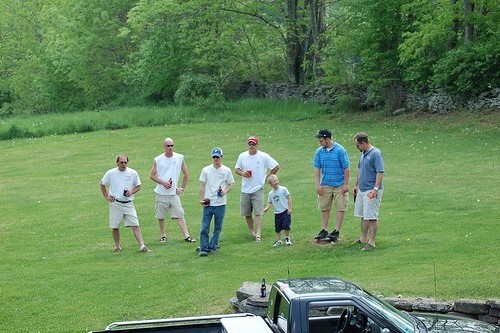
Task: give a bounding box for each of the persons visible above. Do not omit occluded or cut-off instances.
[313,129,350,239]
[263,173,292,246]
[150,138,195,242]
[198,147,235,256]
[236,136,280,241]
[350,132,385,250]
[100,155,147,252]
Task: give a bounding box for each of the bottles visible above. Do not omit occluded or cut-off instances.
[218,187,222,196]
[354,189,357,202]
[260,279,266,297]
[169,178,172,188]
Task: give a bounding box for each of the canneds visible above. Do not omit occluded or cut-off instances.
[247,169,252,177]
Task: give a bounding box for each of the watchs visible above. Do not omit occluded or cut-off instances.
[181,188,184,191]
[374,187,379,190]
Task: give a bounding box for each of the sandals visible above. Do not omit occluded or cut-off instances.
[185,236,196,242]
[160,236,166,243]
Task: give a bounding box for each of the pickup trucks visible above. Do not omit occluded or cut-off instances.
[87,277,500,333]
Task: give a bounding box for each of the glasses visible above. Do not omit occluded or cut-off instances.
[213,156,219,158]
[167,145,174,147]
[248,143,256,146]
[120,161,128,164]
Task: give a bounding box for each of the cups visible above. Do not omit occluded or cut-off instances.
[123,190,128,196]
[247,170,251,177]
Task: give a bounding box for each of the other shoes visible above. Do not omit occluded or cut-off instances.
[273,239,283,247]
[285,237,291,245]
[314,229,328,239]
[360,241,376,251]
[141,247,150,252]
[350,239,363,244]
[328,229,340,239]
[113,247,121,253]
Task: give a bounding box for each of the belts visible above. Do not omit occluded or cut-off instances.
[116,200,131,203]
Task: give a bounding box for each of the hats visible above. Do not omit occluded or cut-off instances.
[247,136,258,145]
[313,129,331,138]
[211,148,223,157]
